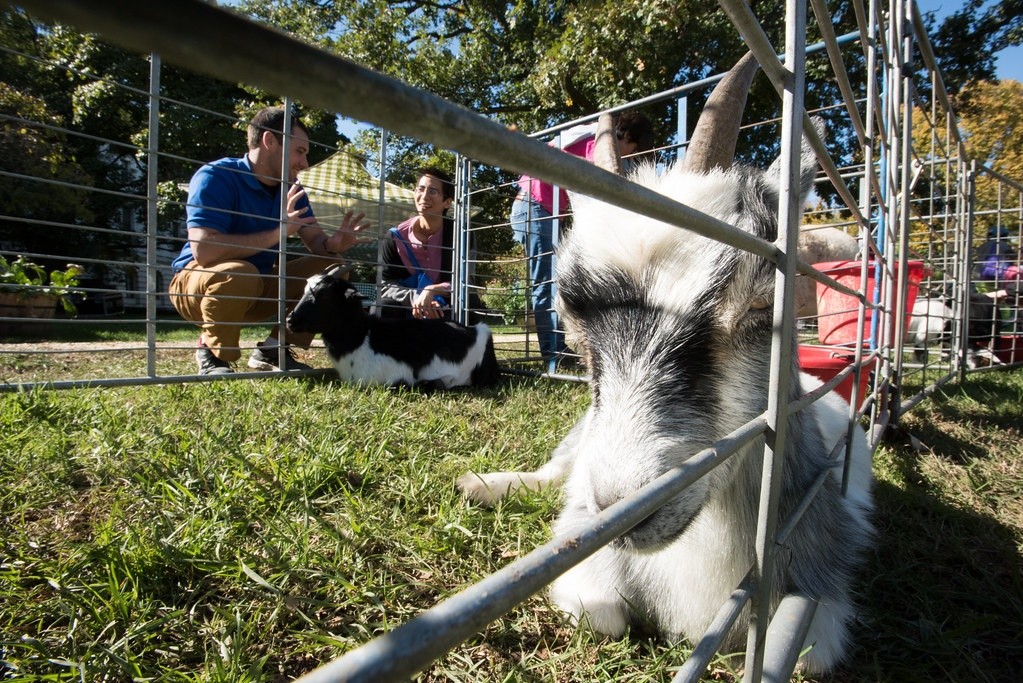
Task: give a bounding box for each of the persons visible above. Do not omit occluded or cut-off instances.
[974,226,1022,301]
[510,112,650,361]
[370,168,482,325]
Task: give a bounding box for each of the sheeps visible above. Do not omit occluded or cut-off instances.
[456,50,1007,679]
[286,263,505,395]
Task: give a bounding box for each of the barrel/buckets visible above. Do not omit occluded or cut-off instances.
[810,260,932,350]
[797,344,876,418]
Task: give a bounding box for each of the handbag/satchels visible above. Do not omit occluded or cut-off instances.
[397,271,447,307]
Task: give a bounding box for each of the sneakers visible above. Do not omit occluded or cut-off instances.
[246,341,312,372]
[195,336,235,375]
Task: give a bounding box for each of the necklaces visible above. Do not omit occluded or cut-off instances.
[169,108,373,376]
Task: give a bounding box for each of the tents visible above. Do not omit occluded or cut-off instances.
[159,143,484,249]
